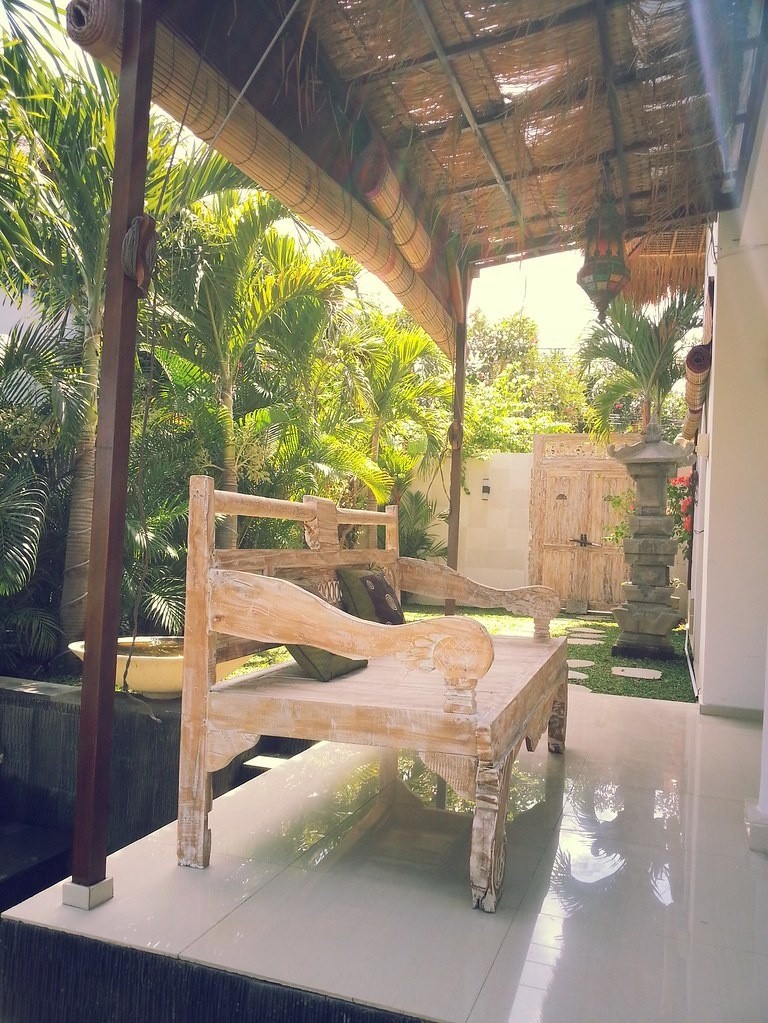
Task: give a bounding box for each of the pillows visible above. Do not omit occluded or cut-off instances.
[334,568,408,626]
[283,585,368,681]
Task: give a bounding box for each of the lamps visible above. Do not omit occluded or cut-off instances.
[575,159,631,324]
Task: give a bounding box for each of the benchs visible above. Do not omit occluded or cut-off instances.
[176,475,569,914]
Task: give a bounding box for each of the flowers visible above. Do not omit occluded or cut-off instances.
[665,475,694,560]
